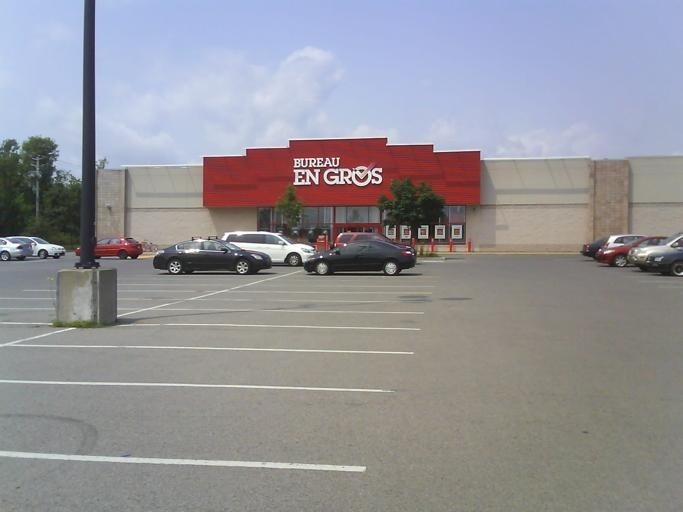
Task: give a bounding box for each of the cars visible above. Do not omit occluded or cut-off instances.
[75,236,143,261]
[0,238,33,261]
[304,241,416,277]
[152,238,272,276]
[580,234,682,278]
[5,236,66,259]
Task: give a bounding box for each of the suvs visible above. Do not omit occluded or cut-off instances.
[333,232,415,254]
[221,230,316,267]
